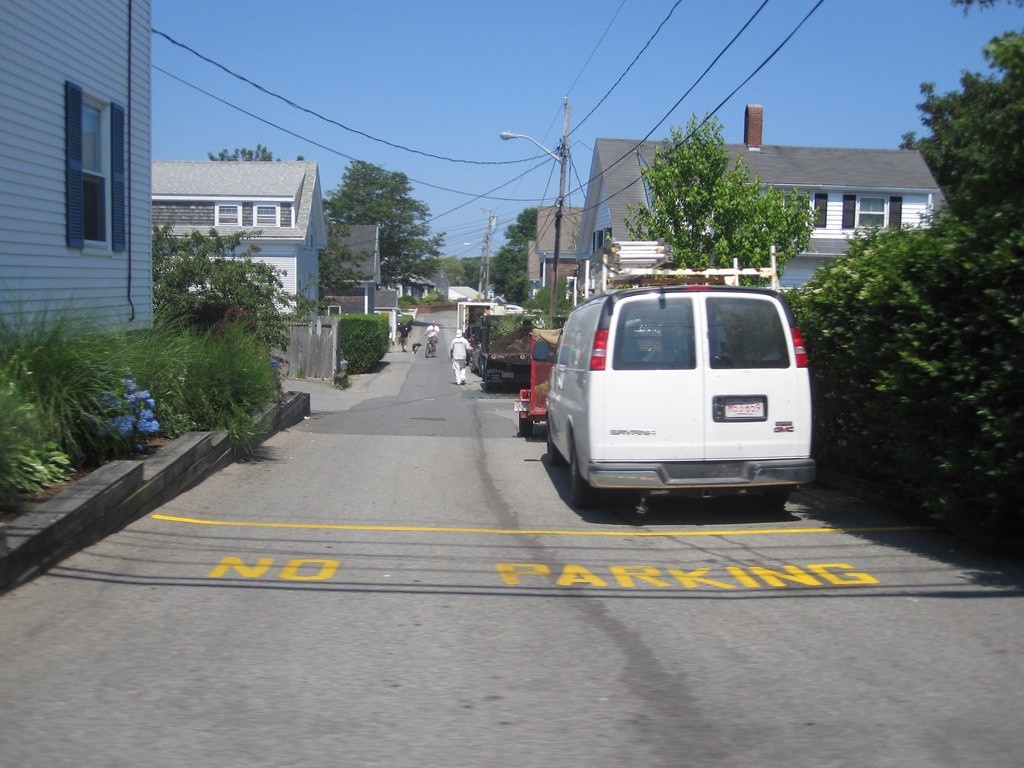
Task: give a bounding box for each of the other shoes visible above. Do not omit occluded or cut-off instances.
[402,349,406,352]
[461,379,465,385]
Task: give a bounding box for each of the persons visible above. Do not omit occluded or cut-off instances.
[424,321,439,353]
[449,329,474,384]
[398,321,412,351]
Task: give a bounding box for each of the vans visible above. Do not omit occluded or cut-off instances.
[531,283,815,516]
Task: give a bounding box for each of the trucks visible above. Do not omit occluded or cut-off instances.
[457,301,545,394]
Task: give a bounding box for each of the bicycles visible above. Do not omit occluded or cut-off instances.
[423,333,438,358]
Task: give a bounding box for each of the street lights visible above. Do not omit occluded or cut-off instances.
[498,130,568,329]
[464,243,490,301]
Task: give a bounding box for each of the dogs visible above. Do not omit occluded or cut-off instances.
[412,343,422,355]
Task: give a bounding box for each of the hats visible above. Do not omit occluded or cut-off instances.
[456,329,462,338]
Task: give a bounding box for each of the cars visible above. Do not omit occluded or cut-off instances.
[515,328,566,437]
